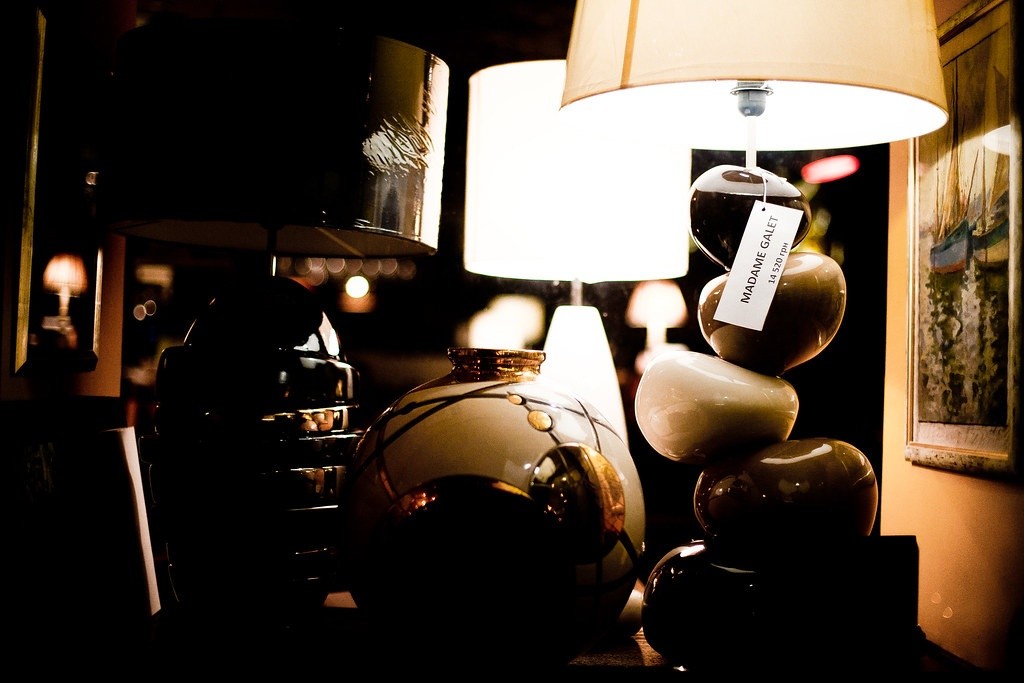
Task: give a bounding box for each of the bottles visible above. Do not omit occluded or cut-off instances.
[139,261,646,671]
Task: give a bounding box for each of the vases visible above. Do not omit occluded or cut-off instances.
[342,349,645,682]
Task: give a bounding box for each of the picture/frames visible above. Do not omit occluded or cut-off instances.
[907,1,1024,482]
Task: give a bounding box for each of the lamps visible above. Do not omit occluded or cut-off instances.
[465,59,694,453]
[90,14,453,682]
[555,3,948,176]
